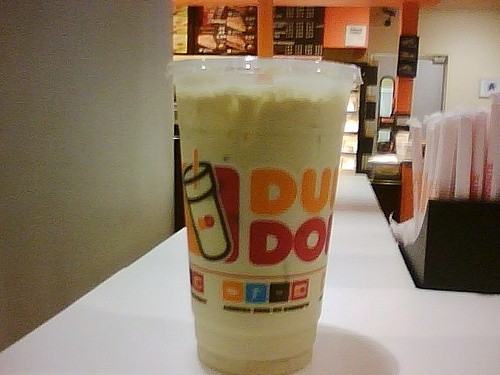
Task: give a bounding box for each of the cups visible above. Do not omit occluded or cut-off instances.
[170,57,357,375]
